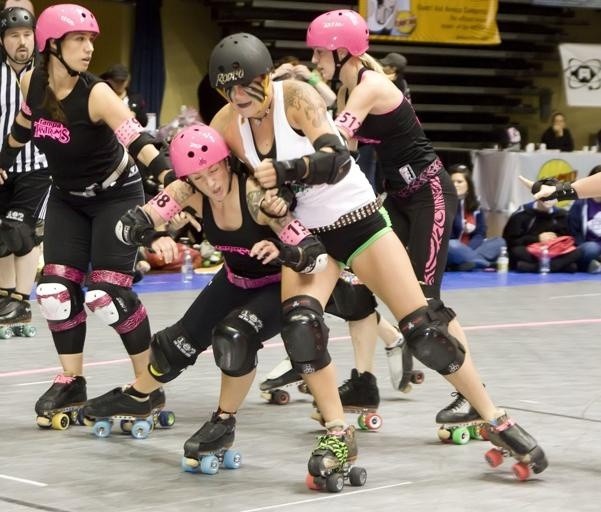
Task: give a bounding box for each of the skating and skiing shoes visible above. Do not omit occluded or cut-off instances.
[305,423,368,493]
[181,413,241,475]
[1,289,35,338]
[480,409,549,481]
[258,355,313,405]
[434,381,495,445]
[382,324,424,395]
[310,369,382,431]
[31,373,175,441]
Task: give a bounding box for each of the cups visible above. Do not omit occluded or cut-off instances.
[581,145,599,152]
[525,142,547,153]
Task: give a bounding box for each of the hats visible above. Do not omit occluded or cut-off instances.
[107,63,129,81]
[379,53,407,68]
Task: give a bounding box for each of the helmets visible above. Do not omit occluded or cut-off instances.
[305,8,372,56]
[0,3,99,54]
[167,122,233,178]
[207,32,273,89]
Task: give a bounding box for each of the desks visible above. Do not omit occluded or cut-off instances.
[466,145,601,243]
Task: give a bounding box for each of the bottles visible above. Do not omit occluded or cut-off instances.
[181,250,194,284]
[539,247,550,273]
[496,245,510,273]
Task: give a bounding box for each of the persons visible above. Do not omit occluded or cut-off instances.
[99,67,148,126]
[0,6,53,326]
[0,3,178,423]
[380,52,413,111]
[207,34,543,478]
[303,10,486,424]
[84,124,329,458]
